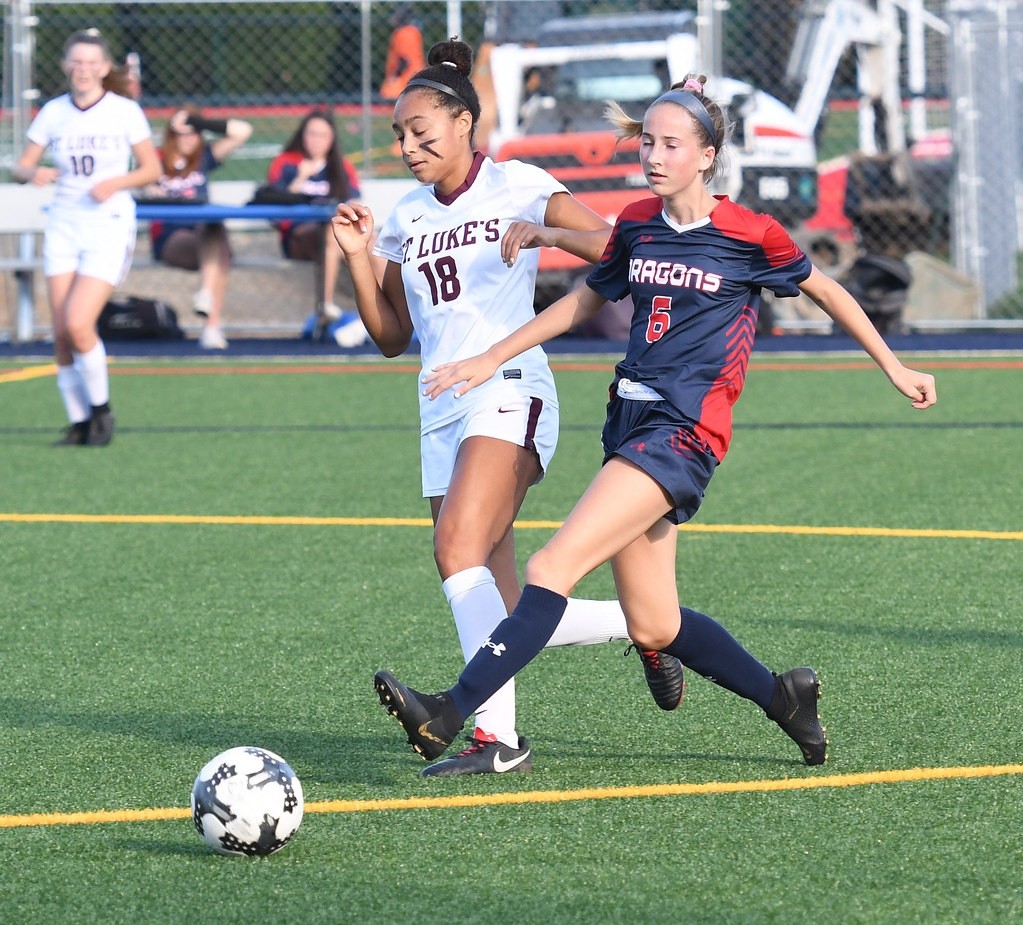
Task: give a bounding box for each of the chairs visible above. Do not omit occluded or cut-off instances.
[0,183,54,344]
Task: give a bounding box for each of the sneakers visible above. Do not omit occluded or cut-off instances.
[86,402,113,445]
[625,643,683,711]
[60,420,89,446]
[373,671,464,761]
[421,728,531,778]
[772,668,829,766]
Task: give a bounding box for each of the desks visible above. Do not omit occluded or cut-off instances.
[135,205,337,338]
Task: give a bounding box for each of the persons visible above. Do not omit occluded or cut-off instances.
[332,41,686,777]
[374,72,937,768]
[11,30,163,445]
[270,109,385,328]
[382,7,425,156]
[138,110,253,349]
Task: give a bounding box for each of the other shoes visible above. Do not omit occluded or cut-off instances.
[198,327,230,350]
[192,289,213,318]
[318,304,341,327]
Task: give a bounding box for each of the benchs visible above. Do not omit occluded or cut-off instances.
[133,258,314,335]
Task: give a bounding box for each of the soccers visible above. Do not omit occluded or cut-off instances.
[189,745,306,859]
